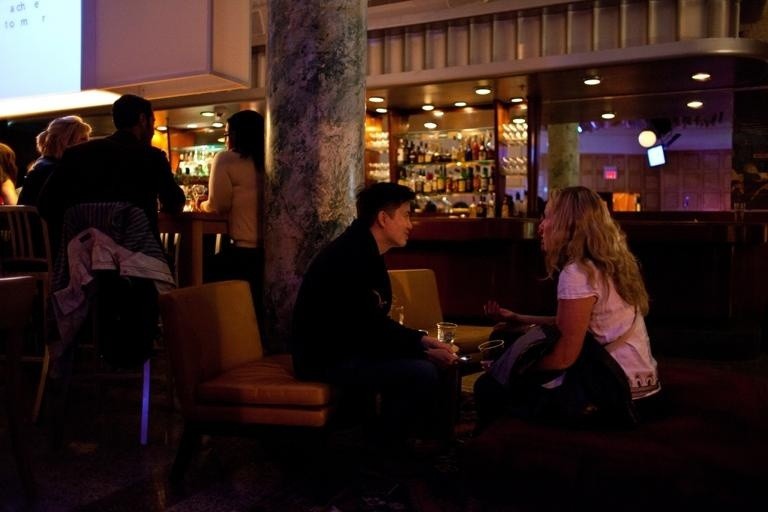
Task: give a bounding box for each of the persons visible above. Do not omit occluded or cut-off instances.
[474,185,663,420]
[186,109,266,291]
[40,93,187,369]
[286,180,467,460]
[14,112,93,204]
[0,143,19,208]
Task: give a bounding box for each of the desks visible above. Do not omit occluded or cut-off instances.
[158,211,227,288]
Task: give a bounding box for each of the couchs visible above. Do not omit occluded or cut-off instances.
[164,266,496,432]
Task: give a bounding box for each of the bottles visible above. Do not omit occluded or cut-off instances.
[425,145,464,162]
[473,137,478,160]
[397,139,405,163]
[476,196,486,217]
[486,132,496,160]
[513,192,520,215]
[636,197,642,212]
[437,166,445,194]
[406,166,437,194]
[473,165,480,191]
[502,196,509,218]
[480,168,487,192]
[177,151,215,176]
[683,196,689,209]
[478,136,487,160]
[465,144,472,160]
[417,142,425,162]
[488,197,496,218]
[470,196,477,218]
[446,169,466,193]
[409,141,417,162]
[466,167,473,192]
[397,170,405,186]
[488,167,495,191]
[520,190,528,217]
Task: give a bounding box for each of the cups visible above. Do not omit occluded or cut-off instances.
[436,322,458,347]
[477,339,505,361]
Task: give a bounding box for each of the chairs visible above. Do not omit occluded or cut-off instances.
[0,201,57,417]
